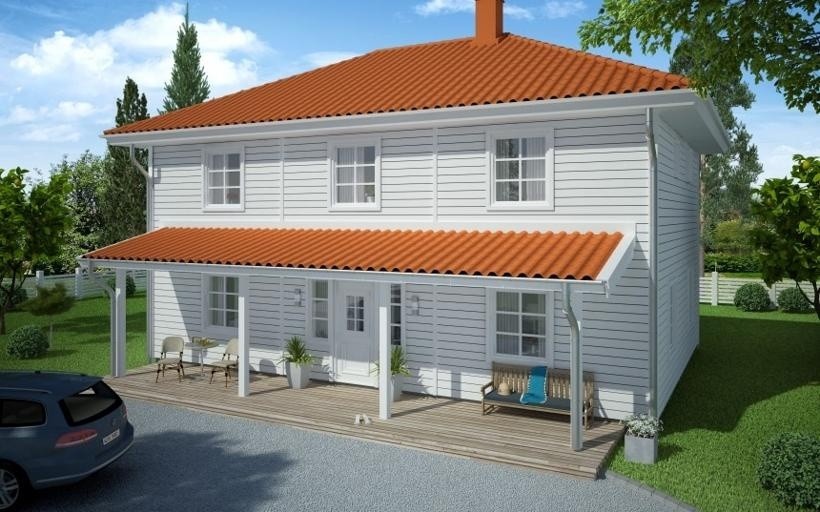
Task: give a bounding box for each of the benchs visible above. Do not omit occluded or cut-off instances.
[480,361,595,432]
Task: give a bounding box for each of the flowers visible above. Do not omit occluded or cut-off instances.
[626,413,664,439]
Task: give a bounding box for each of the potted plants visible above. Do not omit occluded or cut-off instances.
[369,345,409,401]
[276,335,310,390]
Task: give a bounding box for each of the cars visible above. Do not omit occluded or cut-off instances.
[0,371,134,512]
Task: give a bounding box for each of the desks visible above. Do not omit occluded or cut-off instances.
[184,342,219,377]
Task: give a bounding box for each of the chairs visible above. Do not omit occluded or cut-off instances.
[155,337,184,383]
[209,338,239,388]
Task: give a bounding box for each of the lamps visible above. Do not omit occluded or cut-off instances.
[411,297,419,316]
[294,289,301,307]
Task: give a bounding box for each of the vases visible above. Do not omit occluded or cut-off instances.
[624,434,658,465]
